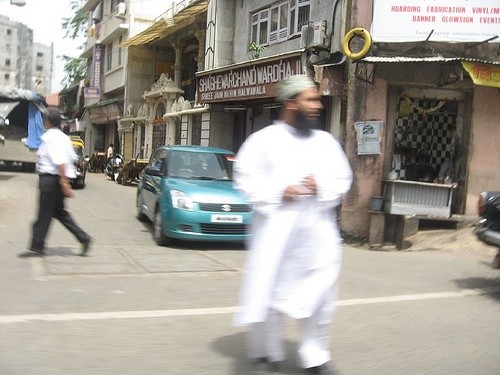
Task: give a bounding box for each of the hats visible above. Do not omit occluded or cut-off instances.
[273,73,316,101]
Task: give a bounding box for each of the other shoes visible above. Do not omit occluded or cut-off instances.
[253,358,279,375]
[81,236,93,257]
[17,249,44,258]
[305,362,336,375]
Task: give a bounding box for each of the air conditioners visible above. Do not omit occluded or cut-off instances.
[300,21,328,49]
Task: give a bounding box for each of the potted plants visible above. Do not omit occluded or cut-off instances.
[249,42,266,59]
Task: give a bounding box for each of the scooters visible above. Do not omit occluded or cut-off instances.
[103,154,125,181]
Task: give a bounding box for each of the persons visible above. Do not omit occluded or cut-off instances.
[16,107,91,256]
[108,144,122,172]
[232,73,353,375]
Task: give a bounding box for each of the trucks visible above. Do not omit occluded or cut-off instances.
[0,85,49,173]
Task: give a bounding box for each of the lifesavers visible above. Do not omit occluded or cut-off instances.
[342,27,373,60]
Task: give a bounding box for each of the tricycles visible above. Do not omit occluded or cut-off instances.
[66,135,86,190]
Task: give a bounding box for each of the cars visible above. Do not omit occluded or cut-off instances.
[134,144,257,251]
